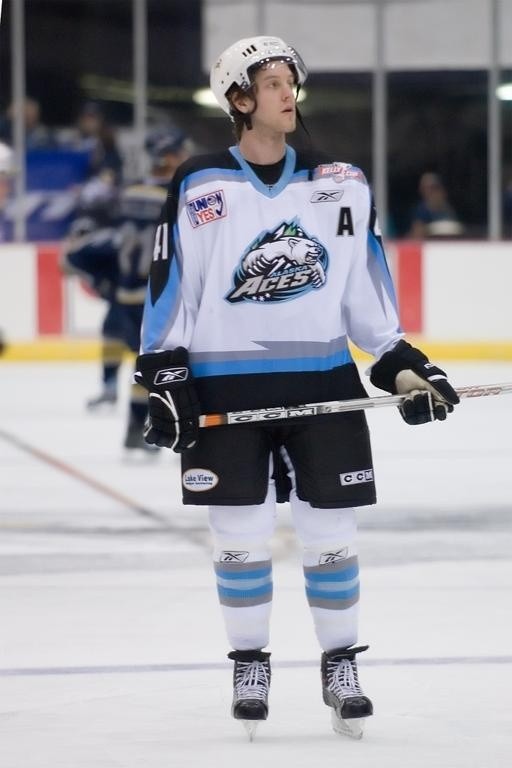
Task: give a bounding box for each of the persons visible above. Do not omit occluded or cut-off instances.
[3,69,512,417]
[138,35,458,726]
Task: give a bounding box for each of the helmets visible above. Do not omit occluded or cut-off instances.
[211,35,305,115]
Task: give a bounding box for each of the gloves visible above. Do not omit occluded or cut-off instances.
[134,347,203,454]
[369,339,458,425]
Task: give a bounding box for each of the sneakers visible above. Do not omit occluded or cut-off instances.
[229,651,272,720]
[321,645,373,719]
